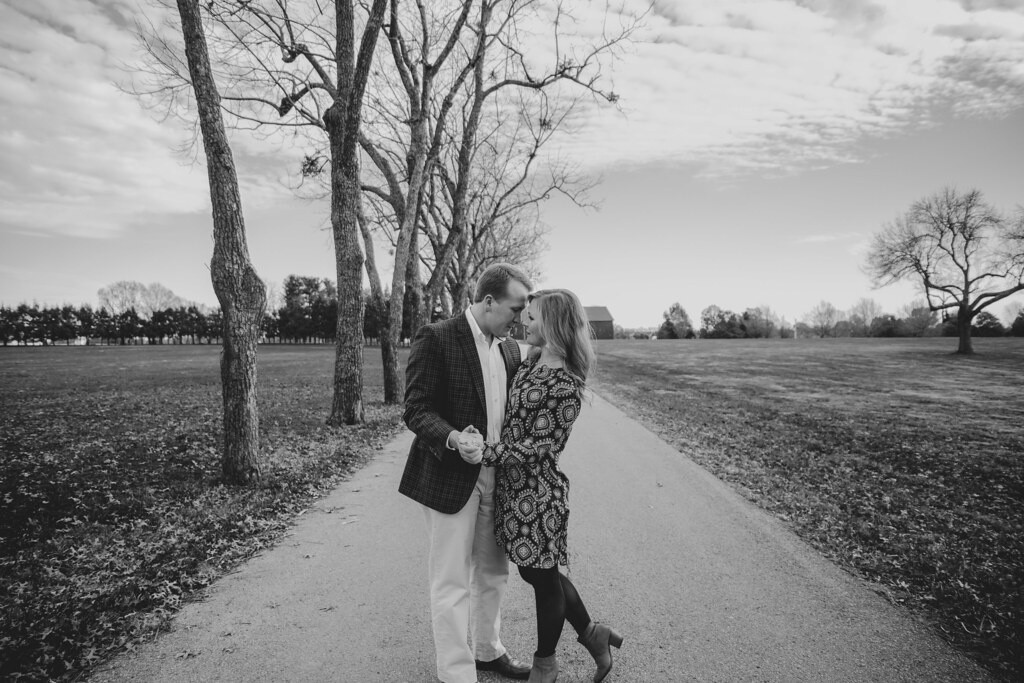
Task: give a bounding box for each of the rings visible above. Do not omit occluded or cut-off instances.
[473,438,475,443]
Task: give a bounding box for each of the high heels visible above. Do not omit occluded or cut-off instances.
[527,651,558,683]
[577,622,624,683]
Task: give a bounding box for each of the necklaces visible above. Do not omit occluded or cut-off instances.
[534,360,560,364]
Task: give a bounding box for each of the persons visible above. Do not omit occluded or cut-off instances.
[398,262,533,683]
[458,288,624,683]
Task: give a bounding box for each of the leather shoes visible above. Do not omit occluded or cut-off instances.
[476,653,532,679]
